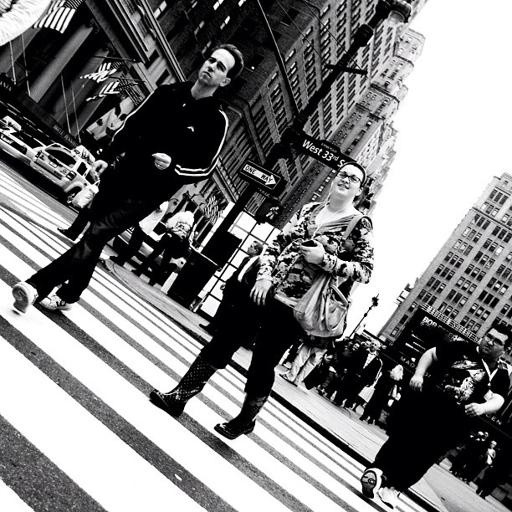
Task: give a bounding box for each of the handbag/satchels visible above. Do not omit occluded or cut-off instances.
[173,239,190,260]
[485,455,493,465]
[293,273,349,339]
[388,385,397,399]
[71,185,100,210]
[359,385,375,403]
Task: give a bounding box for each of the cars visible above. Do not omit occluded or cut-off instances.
[0,114,187,273]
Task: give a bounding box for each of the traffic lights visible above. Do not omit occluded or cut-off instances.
[256,200,283,226]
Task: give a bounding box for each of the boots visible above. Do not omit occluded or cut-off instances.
[150,353,218,418]
[214,394,271,438]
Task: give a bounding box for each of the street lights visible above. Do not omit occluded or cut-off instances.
[346,293,379,338]
[166,0,412,309]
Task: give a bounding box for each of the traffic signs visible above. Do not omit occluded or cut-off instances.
[240,159,288,199]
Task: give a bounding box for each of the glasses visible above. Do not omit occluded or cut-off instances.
[336,171,361,184]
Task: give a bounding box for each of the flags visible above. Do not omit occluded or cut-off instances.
[31,0,85,34]
[85,80,136,103]
[80,59,127,83]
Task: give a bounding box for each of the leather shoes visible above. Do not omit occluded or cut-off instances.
[199,323,207,331]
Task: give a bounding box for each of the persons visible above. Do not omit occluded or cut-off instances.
[11,43,244,314]
[360,323,511,508]
[109,197,170,265]
[87,92,136,141]
[280,310,406,425]
[131,209,195,287]
[447,426,511,500]
[150,160,375,440]
[0,0,50,46]
[58,168,113,240]
[199,240,263,336]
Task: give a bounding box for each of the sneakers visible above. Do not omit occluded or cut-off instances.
[360,466,400,509]
[11,281,38,314]
[132,268,155,286]
[110,253,125,266]
[41,292,73,310]
[280,373,298,386]
[57,227,75,240]
